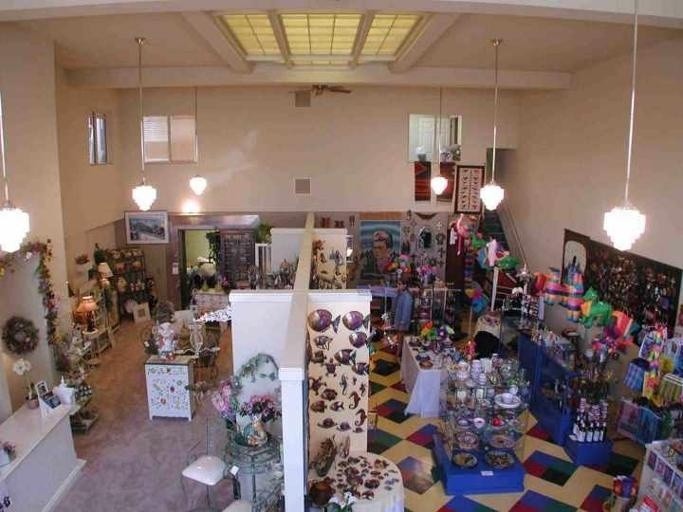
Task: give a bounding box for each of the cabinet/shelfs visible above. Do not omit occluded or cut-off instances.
[87,248,150,333]
[500,310,578,445]
[81,293,113,357]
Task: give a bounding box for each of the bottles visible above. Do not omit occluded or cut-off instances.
[572,415,607,444]
[456,360,471,382]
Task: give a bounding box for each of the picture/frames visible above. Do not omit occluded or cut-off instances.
[131,303,152,324]
[123,210,171,247]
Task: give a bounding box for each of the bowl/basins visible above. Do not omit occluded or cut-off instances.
[473,417,485,429]
[501,393,514,404]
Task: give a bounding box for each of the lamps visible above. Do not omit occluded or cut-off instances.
[0,95,31,255]
[428,1,505,214]
[601,2,647,254]
[97,261,114,285]
[76,295,99,331]
[127,34,209,213]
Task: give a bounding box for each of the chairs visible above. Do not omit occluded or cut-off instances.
[174,415,284,512]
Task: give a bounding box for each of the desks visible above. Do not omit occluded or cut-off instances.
[398,333,468,421]
[307,450,404,512]
[143,351,197,421]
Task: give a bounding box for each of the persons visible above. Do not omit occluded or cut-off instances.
[372,230,398,274]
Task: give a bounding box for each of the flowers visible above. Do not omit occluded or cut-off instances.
[181,351,281,422]
[188,263,230,287]
[1,312,40,400]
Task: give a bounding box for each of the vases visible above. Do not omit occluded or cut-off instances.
[202,280,222,291]
[26,396,39,410]
[242,415,268,447]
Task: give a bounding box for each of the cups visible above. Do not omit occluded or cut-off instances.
[478,373,487,386]
